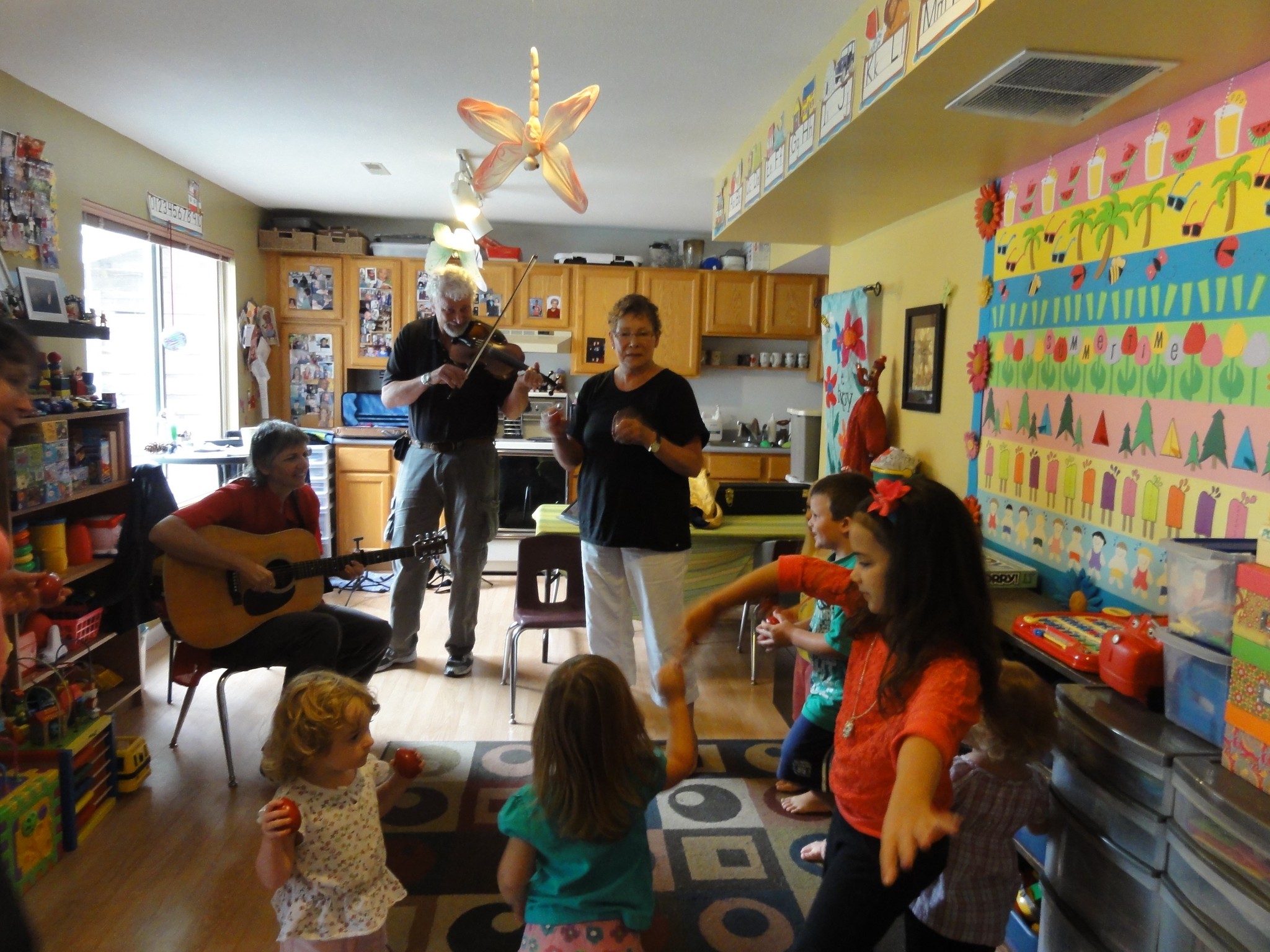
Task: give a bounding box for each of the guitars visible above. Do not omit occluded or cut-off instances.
[151,524,447,649]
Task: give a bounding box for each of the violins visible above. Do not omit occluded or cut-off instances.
[447,322,565,396]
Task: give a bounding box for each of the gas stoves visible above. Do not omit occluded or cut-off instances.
[494,390,571,449]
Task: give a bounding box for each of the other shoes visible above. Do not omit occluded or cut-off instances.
[374,647,417,673]
[258,749,394,792]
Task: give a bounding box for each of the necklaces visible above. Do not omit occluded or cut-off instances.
[843,627,879,738]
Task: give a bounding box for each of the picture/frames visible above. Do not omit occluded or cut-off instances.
[901,302,945,414]
[17,265,69,324]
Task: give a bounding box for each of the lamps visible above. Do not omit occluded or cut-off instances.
[449,172,480,223]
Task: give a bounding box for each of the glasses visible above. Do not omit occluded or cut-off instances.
[613,331,655,341]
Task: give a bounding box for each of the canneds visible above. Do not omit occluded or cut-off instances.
[749,353,759,367]
[710,350,721,365]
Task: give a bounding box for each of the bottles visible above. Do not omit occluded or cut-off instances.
[767,413,777,442]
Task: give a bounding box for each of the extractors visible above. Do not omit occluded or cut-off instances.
[497,329,573,354]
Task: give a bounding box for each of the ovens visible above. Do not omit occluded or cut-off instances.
[481,451,569,574]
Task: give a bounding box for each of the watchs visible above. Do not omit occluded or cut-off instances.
[421,371,434,387]
[647,431,661,454]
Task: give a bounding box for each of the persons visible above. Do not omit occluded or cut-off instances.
[0,301,74,704]
[471,292,499,318]
[373,269,544,672]
[149,418,394,783]
[533,300,541,316]
[547,298,560,319]
[495,652,699,951]
[252,669,427,951]
[284,266,437,429]
[539,294,711,752]
[669,448,1064,952]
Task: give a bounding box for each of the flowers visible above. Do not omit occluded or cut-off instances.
[963,182,1001,525]
[867,478,911,520]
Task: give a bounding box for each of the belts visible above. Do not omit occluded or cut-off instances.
[410,438,493,454]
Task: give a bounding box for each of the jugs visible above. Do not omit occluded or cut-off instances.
[683,239,705,269]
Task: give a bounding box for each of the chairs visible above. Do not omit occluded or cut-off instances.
[504,538,588,725]
[170,659,262,789]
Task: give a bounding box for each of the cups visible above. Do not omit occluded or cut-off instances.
[796,353,809,368]
[759,352,771,367]
[710,350,722,366]
[784,352,796,368]
[770,352,783,367]
[32,516,69,572]
[702,350,707,365]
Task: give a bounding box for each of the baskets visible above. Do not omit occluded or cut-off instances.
[49,606,103,648]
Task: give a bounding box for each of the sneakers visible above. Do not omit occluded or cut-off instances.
[444,651,474,676]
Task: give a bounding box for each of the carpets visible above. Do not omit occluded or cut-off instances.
[369,740,908,952]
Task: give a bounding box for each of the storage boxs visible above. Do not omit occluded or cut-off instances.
[14,421,74,512]
[1155,540,1269,795]
[259,232,369,257]
[975,548,1038,590]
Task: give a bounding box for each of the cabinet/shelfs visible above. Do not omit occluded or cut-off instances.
[1,322,144,745]
[971,592,1269,952]
[264,257,406,430]
[701,456,789,498]
[308,445,447,570]
[402,257,829,383]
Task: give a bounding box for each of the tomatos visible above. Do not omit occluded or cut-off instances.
[271,796,301,833]
[394,747,421,779]
[36,572,62,603]
[765,616,780,626]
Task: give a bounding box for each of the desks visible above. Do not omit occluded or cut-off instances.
[151,449,249,491]
[530,504,807,685]
